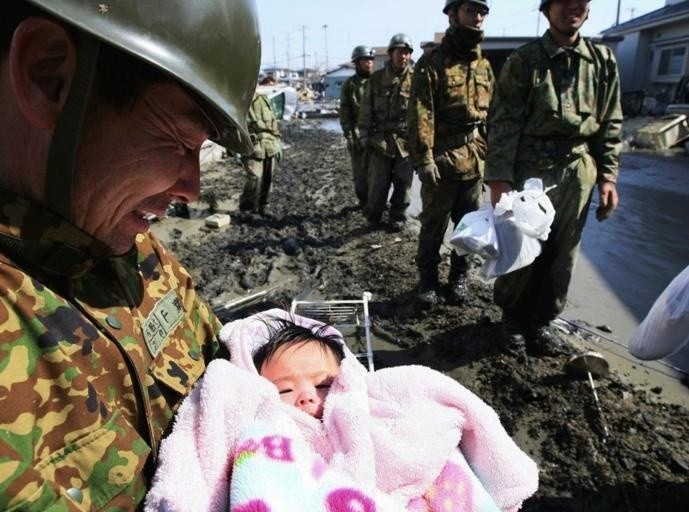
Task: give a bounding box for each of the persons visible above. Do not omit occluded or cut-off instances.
[234,88,284,217]
[404,0,507,311]
[355,31,421,235]
[138,304,545,512]
[338,43,378,208]
[1,0,264,512]
[479,0,622,359]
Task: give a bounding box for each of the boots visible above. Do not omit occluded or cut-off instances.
[499,317,561,354]
[368,211,404,232]
[416,268,469,307]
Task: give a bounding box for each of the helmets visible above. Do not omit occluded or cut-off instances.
[388,34,413,53]
[351,46,375,62]
[28,1,263,156]
[442,0,489,14]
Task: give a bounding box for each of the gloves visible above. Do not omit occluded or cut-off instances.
[596,181,619,221]
[488,181,512,210]
[416,162,440,188]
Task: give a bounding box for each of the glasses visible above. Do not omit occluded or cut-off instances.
[458,6,489,14]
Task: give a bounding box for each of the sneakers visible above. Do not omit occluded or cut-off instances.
[628,265,689,360]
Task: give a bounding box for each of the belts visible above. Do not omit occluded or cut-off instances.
[459,127,480,145]
